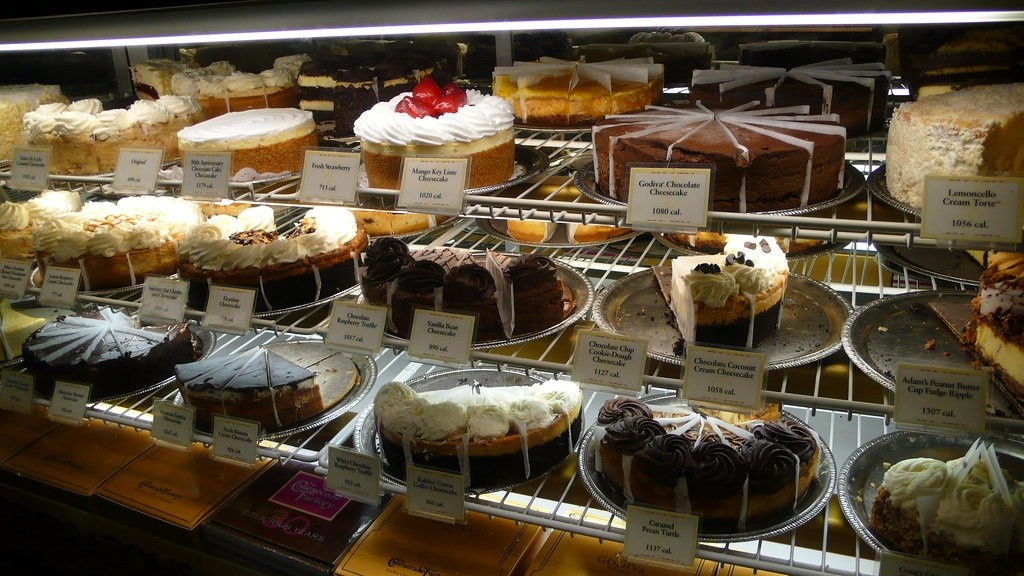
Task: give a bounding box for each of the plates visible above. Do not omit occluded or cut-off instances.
[0,147,1024,555]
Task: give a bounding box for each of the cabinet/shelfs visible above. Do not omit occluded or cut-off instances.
[0,116,1024,576]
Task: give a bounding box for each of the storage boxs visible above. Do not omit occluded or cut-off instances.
[0,408,878,576]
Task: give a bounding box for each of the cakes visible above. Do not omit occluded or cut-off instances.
[0,30,1024,576]
